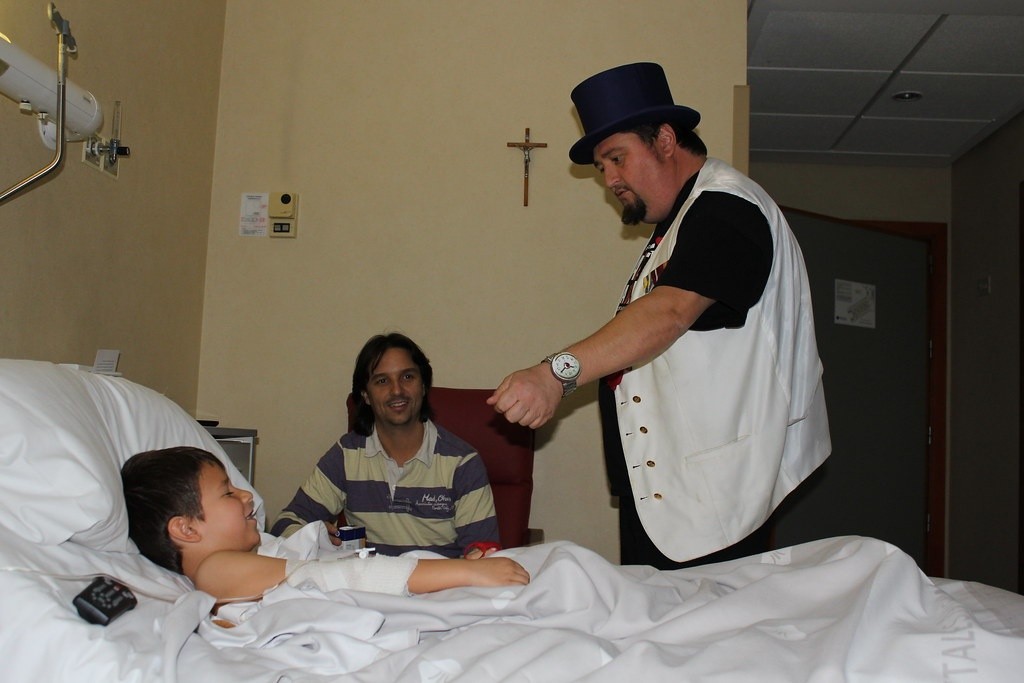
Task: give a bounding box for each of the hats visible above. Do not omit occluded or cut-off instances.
[568,62,701,165]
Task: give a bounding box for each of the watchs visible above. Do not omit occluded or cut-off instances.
[541,350,581,400]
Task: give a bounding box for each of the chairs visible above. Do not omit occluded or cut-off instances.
[347,383,534,548]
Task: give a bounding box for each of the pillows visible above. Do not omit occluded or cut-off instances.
[1,356,267,553]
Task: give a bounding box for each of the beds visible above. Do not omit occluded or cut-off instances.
[0,534,1024,683]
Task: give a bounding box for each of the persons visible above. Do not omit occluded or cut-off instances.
[269,332,499,563]
[491,61,835,575]
[121,447,530,627]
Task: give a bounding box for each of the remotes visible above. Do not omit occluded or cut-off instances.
[72,577,137,626]
